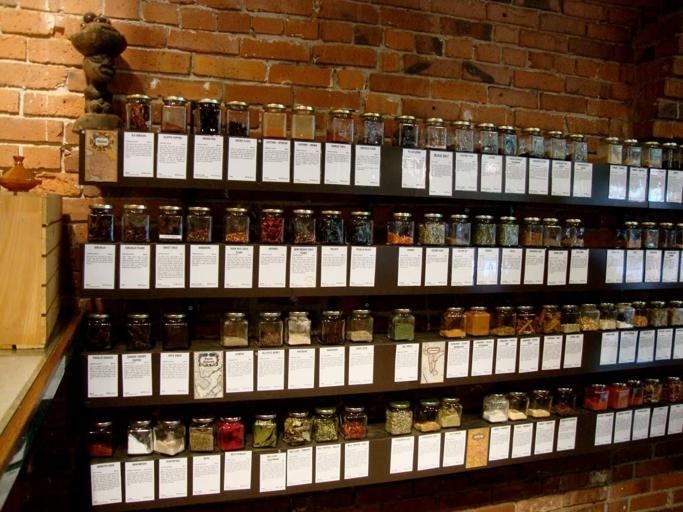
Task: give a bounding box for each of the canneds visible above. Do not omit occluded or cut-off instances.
[125,312,155,351]
[452,121,473,152]
[631,302,649,327]
[89,423,118,458]
[614,303,634,329]
[626,379,644,408]
[553,388,576,417]
[464,306,490,336]
[475,215,496,245]
[339,406,368,440]
[330,109,353,144]
[668,301,683,325]
[284,409,312,444]
[676,224,683,250]
[346,309,374,343]
[157,205,183,244]
[491,307,515,336]
[198,98,222,136]
[584,384,609,411]
[387,212,414,245]
[567,134,588,162]
[599,302,617,329]
[262,103,287,139]
[125,94,152,132]
[607,383,629,410]
[542,218,562,247]
[514,307,540,335]
[223,313,248,346]
[121,204,150,244]
[190,418,214,452]
[658,223,676,250]
[251,415,278,448]
[284,312,311,345]
[665,376,682,403]
[641,222,658,249]
[418,214,447,246]
[215,415,244,451]
[521,217,542,247]
[152,418,184,455]
[388,307,415,342]
[498,125,518,156]
[345,211,374,247]
[87,203,114,243]
[649,302,668,327]
[563,219,583,246]
[258,209,285,245]
[412,400,441,432]
[662,142,679,170]
[622,222,642,249]
[320,210,344,244]
[483,393,509,421]
[441,308,467,339]
[187,207,212,243]
[540,306,561,335]
[438,398,463,428]
[395,116,418,148]
[223,207,250,244]
[677,145,683,170]
[581,306,600,330]
[498,216,519,247]
[518,126,544,158]
[258,312,283,346]
[311,409,338,443]
[359,113,383,145]
[163,313,191,352]
[81,313,113,353]
[162,97,187,134]
[641,142,663,168]
[476,122,498,155]
[561,305,580,334]
[597,137,623,164]
[289,209,316,246]
[385,400,413,435]
[527,389,551,417]
[644,379,661,404]
[424,119,447,149]
[127,422,154,454]
[506,392,527,420]
[291,104,315,141]
[543,131,566,159]
[622,139,642,167]
[225,100,249,137]
[321,311,346,344]
[447,214,471,245]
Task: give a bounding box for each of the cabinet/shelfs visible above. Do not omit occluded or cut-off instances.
[53,178,683,512]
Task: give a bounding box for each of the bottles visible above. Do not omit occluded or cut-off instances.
[0,155,38,190]
